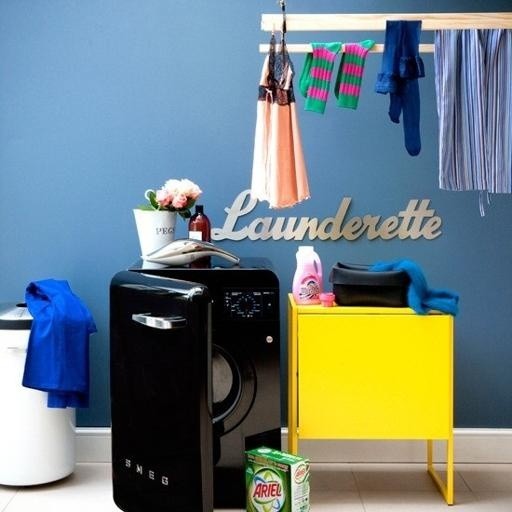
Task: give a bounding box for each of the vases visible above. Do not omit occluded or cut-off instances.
[133,210,177,261]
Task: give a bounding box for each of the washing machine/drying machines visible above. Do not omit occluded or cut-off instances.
[109,256,282,511]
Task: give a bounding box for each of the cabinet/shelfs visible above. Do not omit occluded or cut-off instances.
[287,293,454,505]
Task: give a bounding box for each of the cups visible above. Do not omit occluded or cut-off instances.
[132,209,178,269]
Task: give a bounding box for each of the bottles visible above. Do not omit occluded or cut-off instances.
[188,205,211,265]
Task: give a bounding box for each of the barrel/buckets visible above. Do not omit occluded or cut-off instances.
[1,303,78,486]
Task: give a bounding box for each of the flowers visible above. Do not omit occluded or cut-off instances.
[138,177,202,221]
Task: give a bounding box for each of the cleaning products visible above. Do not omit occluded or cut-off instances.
[291,244,322,305]
[242,447,311,512]
